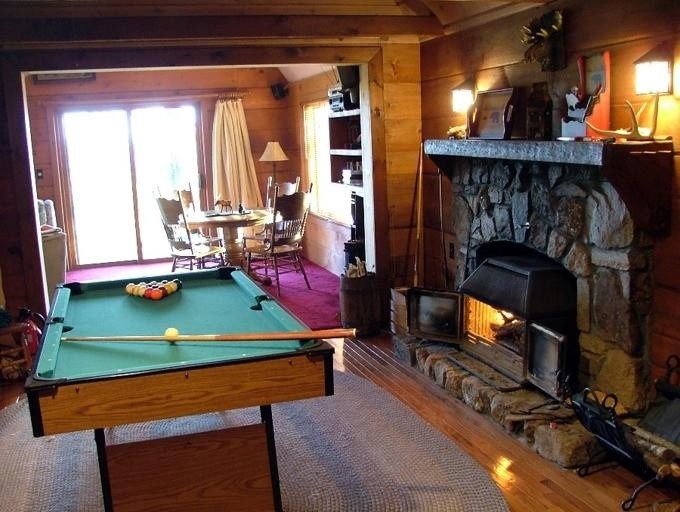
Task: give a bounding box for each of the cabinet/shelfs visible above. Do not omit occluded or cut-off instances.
[327,110,363,188]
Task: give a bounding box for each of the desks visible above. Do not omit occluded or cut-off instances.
[23,265,334,512]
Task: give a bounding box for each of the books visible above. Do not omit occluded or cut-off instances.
[341,160,362,187]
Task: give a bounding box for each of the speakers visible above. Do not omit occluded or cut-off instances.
[337,66,359,90]
[270,84,286,99]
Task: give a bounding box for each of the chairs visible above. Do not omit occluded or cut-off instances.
[150,176,316,297]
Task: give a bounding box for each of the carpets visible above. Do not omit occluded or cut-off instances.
[0,365,511,512]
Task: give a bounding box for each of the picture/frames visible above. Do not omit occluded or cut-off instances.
[466,87,519,140]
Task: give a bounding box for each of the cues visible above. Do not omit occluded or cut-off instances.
[415,142,425,286]
[60,328,355,342]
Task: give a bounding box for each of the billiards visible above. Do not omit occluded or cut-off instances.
[126,279,182,300]
[165,328,177,336]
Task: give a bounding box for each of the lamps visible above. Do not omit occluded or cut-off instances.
[451,78,476,115]
[258,140,290,183]
[632,42,674,97]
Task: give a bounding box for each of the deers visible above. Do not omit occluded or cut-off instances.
[519,8,564,64]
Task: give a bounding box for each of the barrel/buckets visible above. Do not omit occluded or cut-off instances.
[340,273,382,338]
